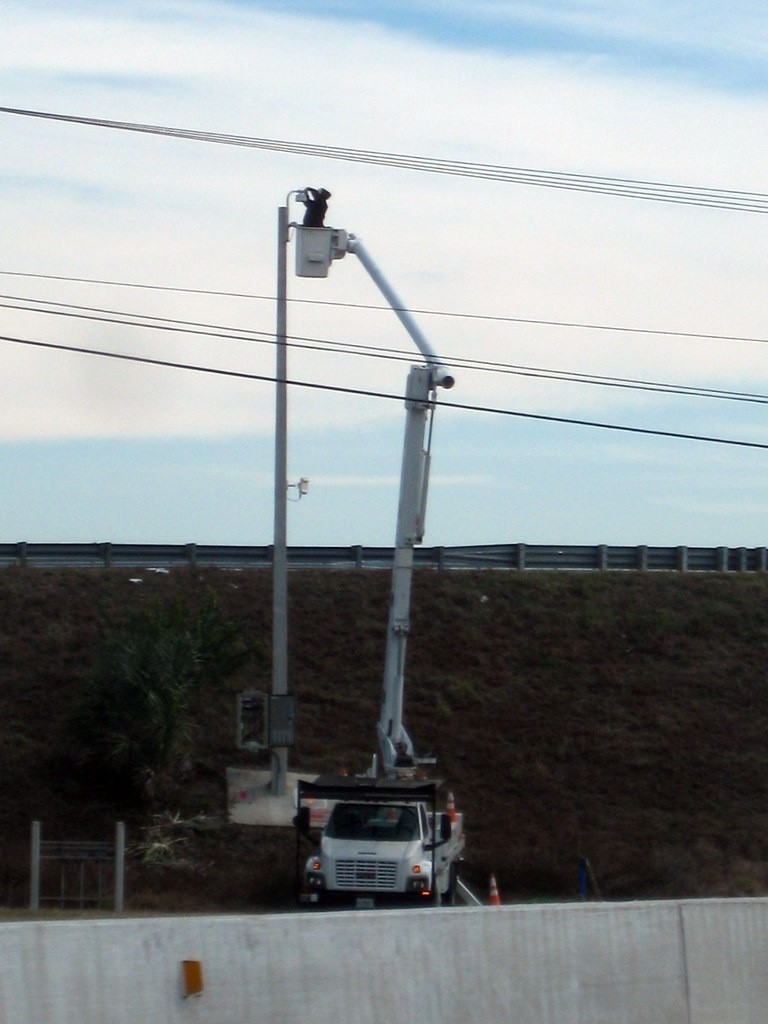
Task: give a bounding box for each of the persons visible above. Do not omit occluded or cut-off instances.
[394,742,414,767]
[303,187,331,227]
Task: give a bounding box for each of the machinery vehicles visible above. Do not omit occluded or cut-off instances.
[230,186,478,908]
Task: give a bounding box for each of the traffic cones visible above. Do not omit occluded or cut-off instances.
[486,877,501,904]
[446,789,457,823]
